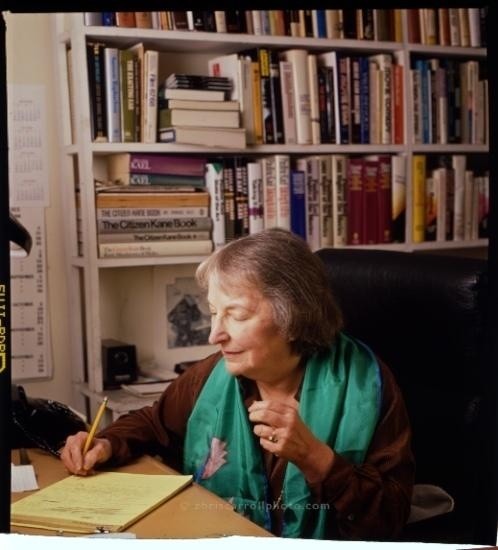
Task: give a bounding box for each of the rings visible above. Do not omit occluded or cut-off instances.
[269,426,277,441]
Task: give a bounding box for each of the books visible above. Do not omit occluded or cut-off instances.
[237,159,248,238]
[413,156,425,243]
[433,169,446,242]
[249,164,265,234]
[438,8,450,46]
[161,88,225,101]
[96,186,210,218]
[136,44,147,142]
[98,217,213,229]
[118,51,136,142]
[297,159,320,250]
[271,69,283,144]
[89,42,106,142]
[146,50,158,144]
[210,56,255,144]
[261,52,273,144]
[161,110,242,129]
[365,155,390,245]
[206,164,225,248]
[252,50,262,142]
[129,173,206,189]
[473,176,492,237]
[281,61,297,144]
[349,160,363,245]
[308,155,334,248]
[280,50,310,144]
[91,9,438,45]
[447,170,454,241]
[452,156,467,242]
[100,233,211,242]
[334,157,348,245]
[257,158,278,229]
[390,156,406,244]
[307,56,322,144]
[425,179,438,241]
[316,52,404,144]
[464,170,473,241]
[277,154,291,231]
[159,126,246,153]
[105,49,121,144]
[99,242,214,260]
[129,156,206,172]
[161,100,239,111]
[223,158,236,242]
[363,161,378,244]
[449,9,461,47]
[412,57,484,143]
[161,74,232,91]
[470,8,481,47]
[291,172,307,238]
[459,9,470,46]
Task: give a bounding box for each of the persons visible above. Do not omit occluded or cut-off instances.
[59,228,413,541]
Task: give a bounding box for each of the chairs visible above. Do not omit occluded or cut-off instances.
[297,248,497,544]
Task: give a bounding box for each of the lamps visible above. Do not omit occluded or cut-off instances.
[9,209,32,257]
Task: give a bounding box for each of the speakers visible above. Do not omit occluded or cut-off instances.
[101,342,138,390]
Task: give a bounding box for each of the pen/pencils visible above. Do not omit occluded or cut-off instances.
[71,394,114,475]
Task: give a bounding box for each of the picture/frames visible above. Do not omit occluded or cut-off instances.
[152,262,223,366]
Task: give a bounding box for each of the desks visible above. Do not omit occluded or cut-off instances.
[10,417,279,539]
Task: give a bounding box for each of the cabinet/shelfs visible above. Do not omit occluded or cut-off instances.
[55,8,496,423]
